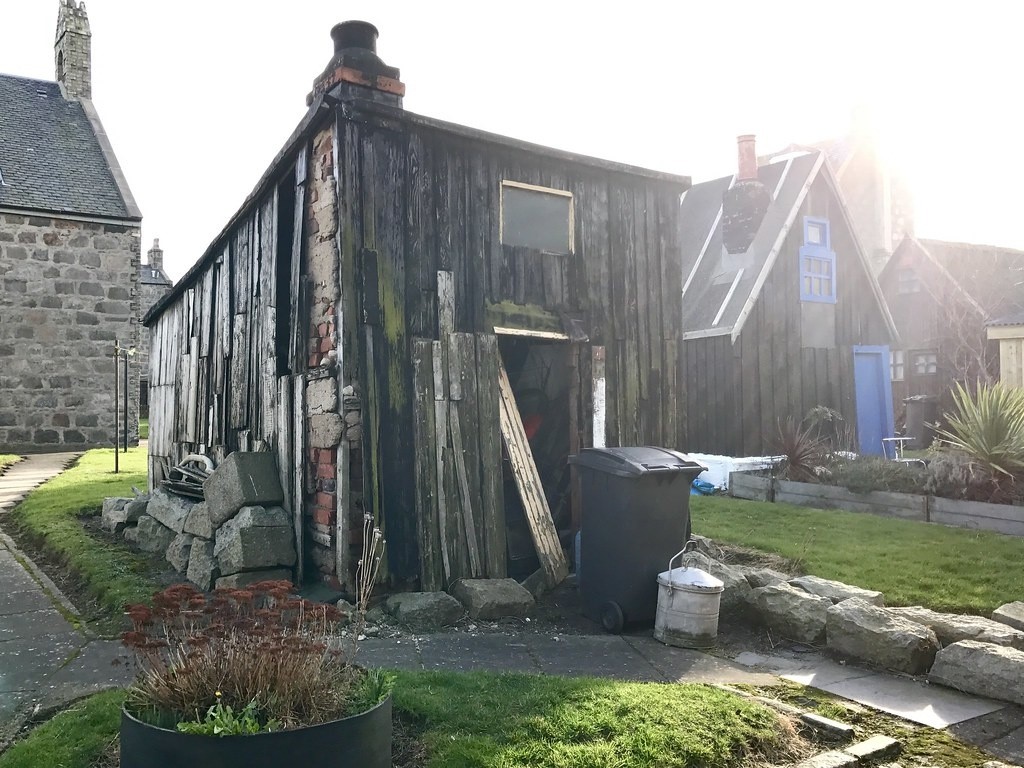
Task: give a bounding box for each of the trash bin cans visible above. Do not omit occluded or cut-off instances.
[565,443,709,636]
[902,394,939,450]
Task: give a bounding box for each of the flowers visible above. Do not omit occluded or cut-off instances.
[119,581,396,738]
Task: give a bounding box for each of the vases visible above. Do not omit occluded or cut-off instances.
[120,665,393,768]
